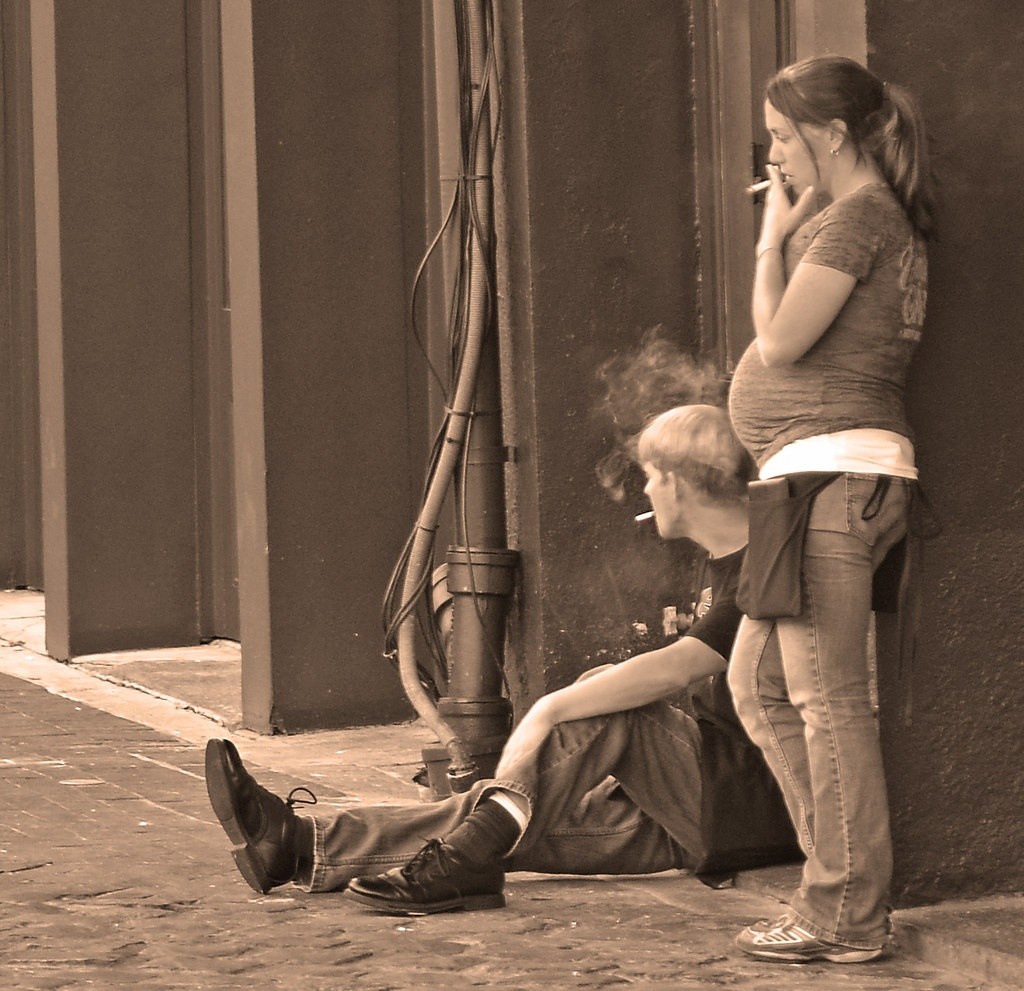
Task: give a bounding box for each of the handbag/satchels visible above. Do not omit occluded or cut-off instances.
[734,473,831,618]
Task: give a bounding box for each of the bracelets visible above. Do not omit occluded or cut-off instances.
[752,244,784,268]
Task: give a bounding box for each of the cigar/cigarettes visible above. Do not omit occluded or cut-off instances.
[745,180,771,194]
[635,510,655,521]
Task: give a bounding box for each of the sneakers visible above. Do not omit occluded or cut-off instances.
[735,916,894,962]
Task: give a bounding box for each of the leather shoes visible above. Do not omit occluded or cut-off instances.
[206,738,318,892]
[345,837,507,914]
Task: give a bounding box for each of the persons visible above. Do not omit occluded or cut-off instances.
[202,405,802,920]
[721,53,931,968]
